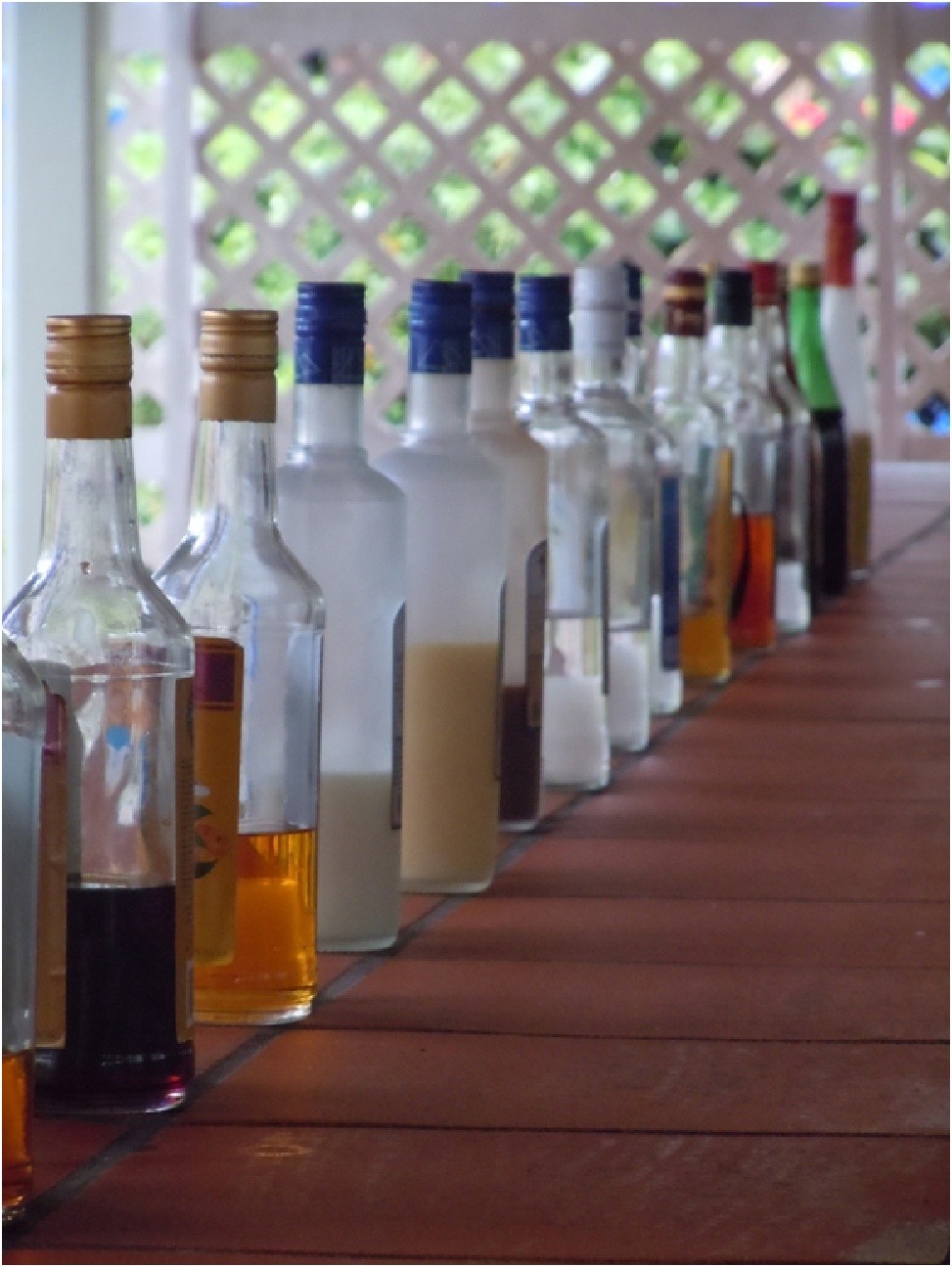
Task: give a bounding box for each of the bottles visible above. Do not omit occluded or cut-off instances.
[625,264,684,715]
[570,261,656,753]
[146,309,324,1029]
[789,260,848,599]
[818,192,872,583]
[515,275,610,796]
[371,278,507,895]
[3,618,49,1236]
[644,269,734,689]
[1,315,198,1110]
[706,271,781,657]
[738,260,813,637]
[275,284,411,955]
[458,270,548,835]
